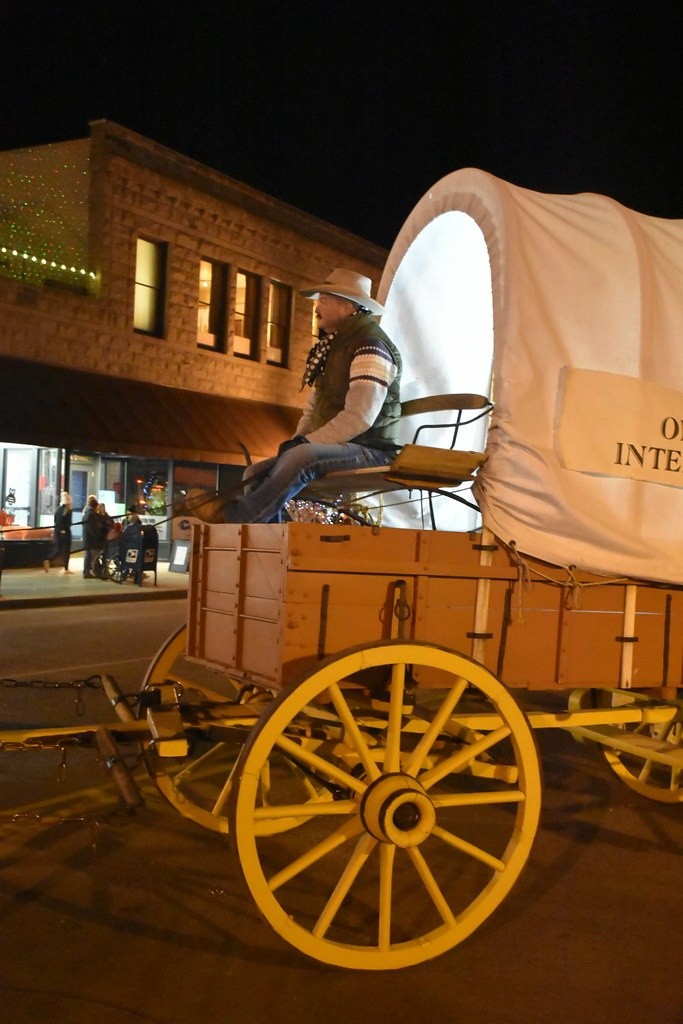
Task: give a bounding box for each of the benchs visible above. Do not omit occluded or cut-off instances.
[290,393,495,530]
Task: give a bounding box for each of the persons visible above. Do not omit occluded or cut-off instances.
[42,491,76,576]
[181,268,404,525]
[80,495,144,578]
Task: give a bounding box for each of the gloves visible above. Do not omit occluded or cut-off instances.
[276,435,307,461]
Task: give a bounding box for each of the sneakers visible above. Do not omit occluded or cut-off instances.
[43,560,50,573]
[140,573,149,580]
[185,488,227,525]
[63,569,75,575]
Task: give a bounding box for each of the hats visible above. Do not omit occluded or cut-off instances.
[128,504,135,512]
[87,495,97,507]
[59,492,71,506]
[295,268,388,316]
